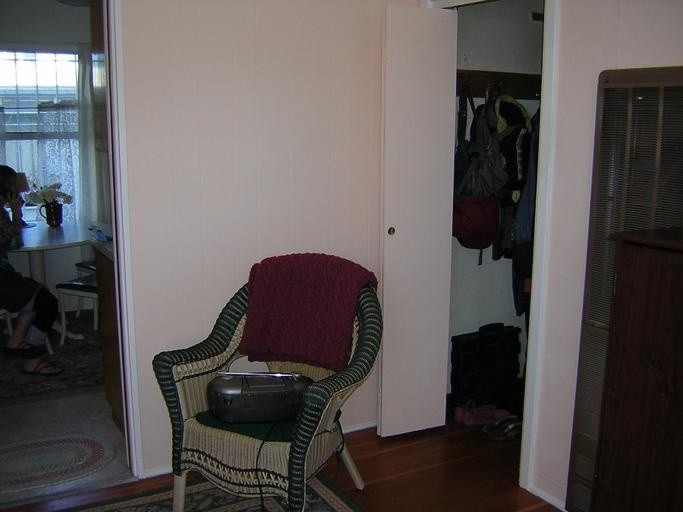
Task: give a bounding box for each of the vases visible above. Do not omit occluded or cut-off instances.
[38,203,64,226]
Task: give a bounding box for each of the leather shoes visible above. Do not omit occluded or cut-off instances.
[455,404,510,428]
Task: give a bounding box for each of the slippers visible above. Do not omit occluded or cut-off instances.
[481,413,522,443]
[4,342,43,359]
[19,358,65,377]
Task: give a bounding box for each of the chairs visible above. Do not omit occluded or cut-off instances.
[1,307,21,335]
[56,272,101,347]
[73,259,98,320]
[151,252,382,511]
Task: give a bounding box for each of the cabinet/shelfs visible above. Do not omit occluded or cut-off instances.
[591,228,683,512]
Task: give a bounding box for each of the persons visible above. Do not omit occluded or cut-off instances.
[0,164,66,377]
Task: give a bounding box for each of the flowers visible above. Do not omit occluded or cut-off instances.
[21,177,73,205]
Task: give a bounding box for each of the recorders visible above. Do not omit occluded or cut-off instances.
[203,370,315,419]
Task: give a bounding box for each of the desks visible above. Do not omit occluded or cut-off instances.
[88,237,124,438]
[5,222,111,354]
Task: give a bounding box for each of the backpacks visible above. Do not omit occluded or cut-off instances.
[470,84,531,204]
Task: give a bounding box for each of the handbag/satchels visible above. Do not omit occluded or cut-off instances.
[457,90,508,197]
[71,333,109,368]
[452,194,500,250]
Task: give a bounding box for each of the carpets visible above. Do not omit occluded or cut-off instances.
[0,383,141,505]
[51,472,365,512]
[0,306,105,398]
